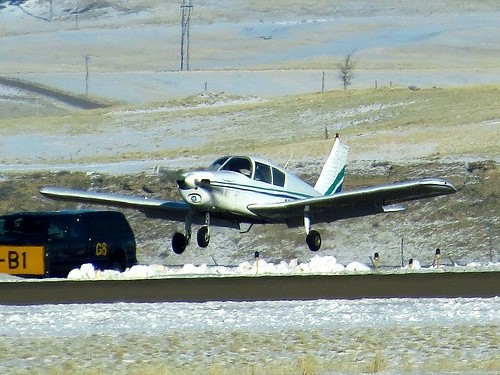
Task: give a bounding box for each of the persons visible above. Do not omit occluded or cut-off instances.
[229,160,241,173]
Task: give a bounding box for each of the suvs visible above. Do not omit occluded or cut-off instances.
[0,209,139,279]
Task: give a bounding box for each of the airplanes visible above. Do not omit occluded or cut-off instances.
[37,132,459,254]
